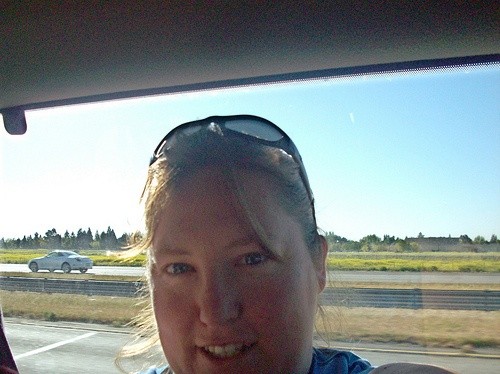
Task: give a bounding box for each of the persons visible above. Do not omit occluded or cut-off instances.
[110,115,453,374]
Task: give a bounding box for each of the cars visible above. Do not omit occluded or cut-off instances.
[28,250,93,273]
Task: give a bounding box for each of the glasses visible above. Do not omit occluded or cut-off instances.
[148,114,318,231]
